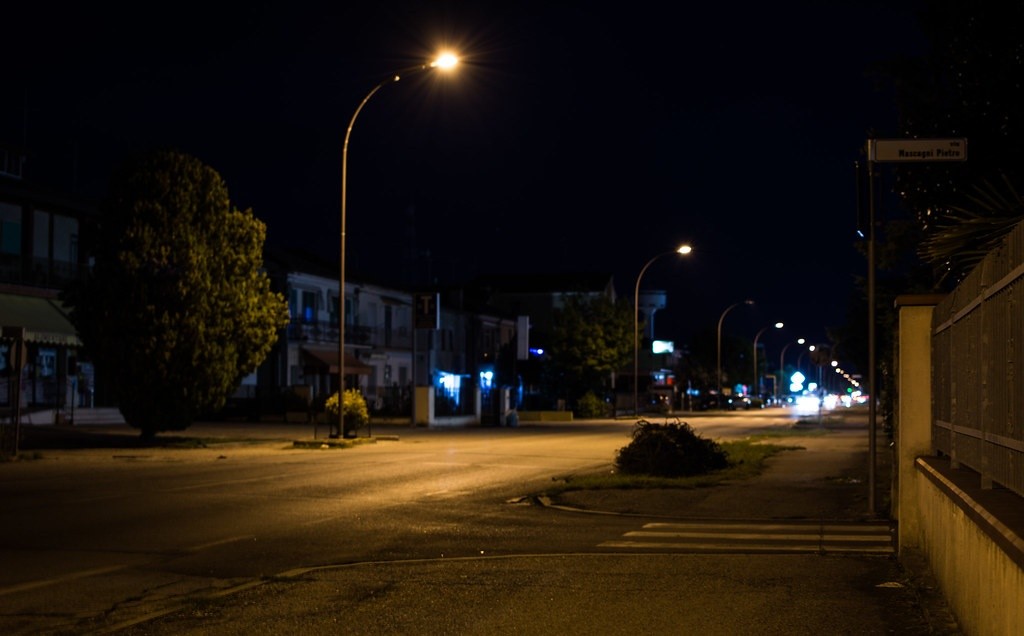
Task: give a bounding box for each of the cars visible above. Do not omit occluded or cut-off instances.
[644,376,869,413]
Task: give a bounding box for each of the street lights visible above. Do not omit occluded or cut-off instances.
[797,345,816,370]
[779,337,806,395]
[335,52,459,439]
[717,298,754,407]
[754,320,783,399]
[632,241,692,418]
